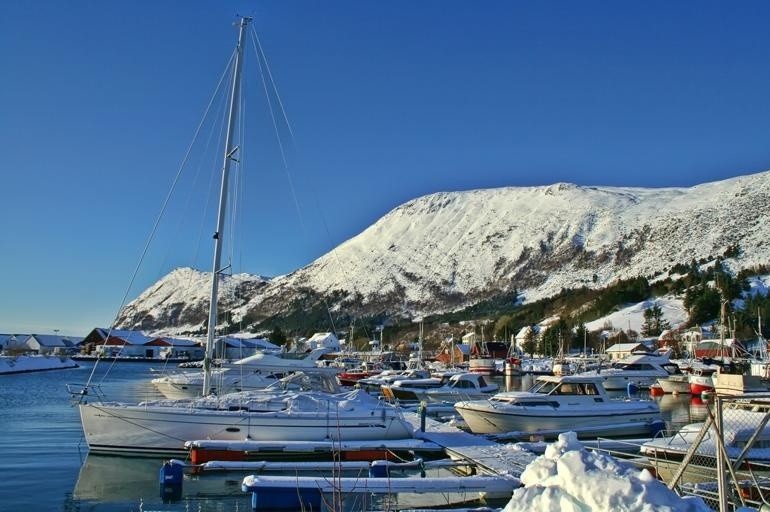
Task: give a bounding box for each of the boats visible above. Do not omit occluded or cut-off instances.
[453,372,667,441]
[638,399,770,512]
[143,344,353,400]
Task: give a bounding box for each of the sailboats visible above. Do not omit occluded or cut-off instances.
[72,13,419,459]
[338,305,769,404]
